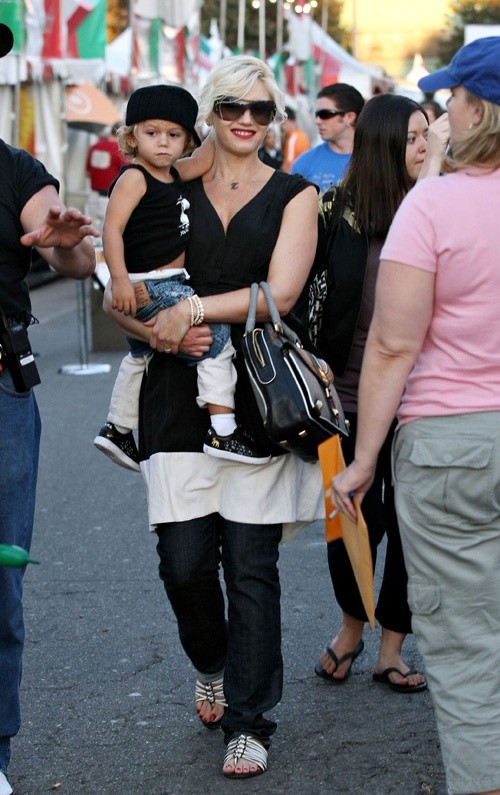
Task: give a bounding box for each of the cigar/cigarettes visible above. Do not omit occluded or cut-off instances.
[328,491,355,519]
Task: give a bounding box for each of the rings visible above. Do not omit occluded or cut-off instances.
[164,348,171,352]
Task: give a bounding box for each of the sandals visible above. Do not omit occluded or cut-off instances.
[223,734,268,778]
[195,677,229,730]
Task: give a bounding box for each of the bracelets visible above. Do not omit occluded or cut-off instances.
[187,293,205,328]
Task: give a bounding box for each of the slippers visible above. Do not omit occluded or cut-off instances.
[373,667,427,693]
[314,638,364,683]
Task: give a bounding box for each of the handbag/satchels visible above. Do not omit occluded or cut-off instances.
[241,282,350,464]
[307,184,345,349]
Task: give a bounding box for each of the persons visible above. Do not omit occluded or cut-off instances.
[0,19,102,795]
[307,94,451,693]
[85,104,314,252]
[94,85,271,474]
[329,35,500,795]
[102,56,351,782]
[287,83,366,203]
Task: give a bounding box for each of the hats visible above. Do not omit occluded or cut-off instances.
[418,36,500,105]
[126,85,202,146]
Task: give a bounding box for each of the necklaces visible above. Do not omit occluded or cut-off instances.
[207,160,264,191]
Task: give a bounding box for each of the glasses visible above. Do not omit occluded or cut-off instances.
[315,109,345,120]
[213,95,277,126]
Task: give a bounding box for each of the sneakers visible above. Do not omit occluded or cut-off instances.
[203,426,272,464]
[93,421,140,472]
